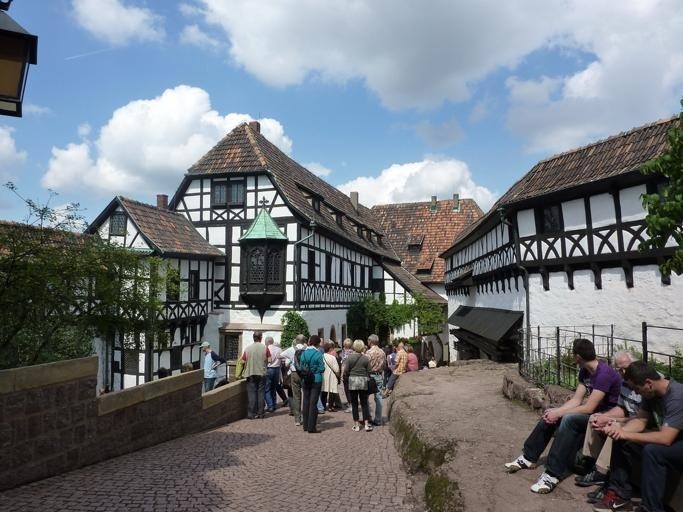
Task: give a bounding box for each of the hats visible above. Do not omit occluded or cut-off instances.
[198,341,210,349]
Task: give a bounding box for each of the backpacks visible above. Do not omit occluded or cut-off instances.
[291,345,303,376]
[300,348,319,384]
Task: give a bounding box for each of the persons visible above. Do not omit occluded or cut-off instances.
[593,362,683,512]
[157,367,169,378]
[198,342,225,392]
[238,332,436,433]
[574,349,665,503]
[182,362,193,373]
[100,383,112,396]
[504,338,622,494]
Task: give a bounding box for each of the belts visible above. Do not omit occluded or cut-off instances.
[370,372,382,375]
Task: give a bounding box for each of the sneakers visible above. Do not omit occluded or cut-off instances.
[303,427,322,433]
[281,399,288,406]
[574,469,607,485]
[503,454,537,474]
[530,471,562,494]
[592,489,633,512]
[381,391,391,398]
[586,485,606,500]
[352,425,373,432]
[263,404,276,412]
[318,406,352,416]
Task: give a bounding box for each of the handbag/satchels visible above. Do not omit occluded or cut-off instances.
[573,447,597,475]
[367,374,380,395]
[235,356,246,379]
